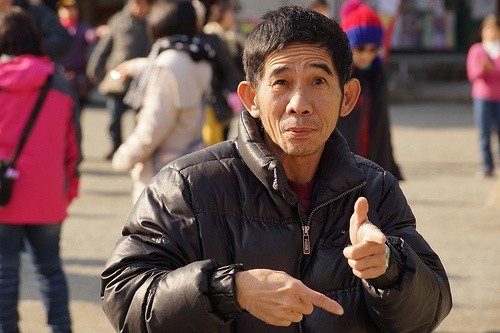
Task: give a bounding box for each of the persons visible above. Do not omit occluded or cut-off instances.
[334,1,405,183]
[466,12,500,177]
[0,8,83,333]
[10,1,252,214]
[99,7,453,333]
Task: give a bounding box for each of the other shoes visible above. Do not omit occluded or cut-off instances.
[483,167,493,176]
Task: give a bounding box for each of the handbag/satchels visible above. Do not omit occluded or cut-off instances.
[0,159,15,205]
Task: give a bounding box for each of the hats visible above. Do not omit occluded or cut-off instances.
[340,0,383,47]
